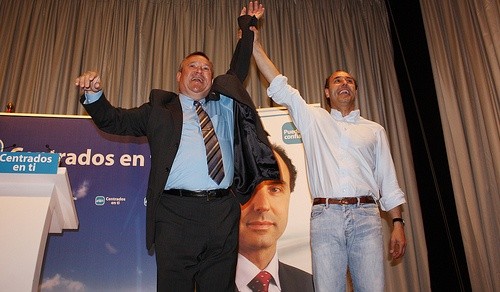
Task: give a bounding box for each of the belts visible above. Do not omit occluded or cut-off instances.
[313,196,376,204]
[164,188,229,198]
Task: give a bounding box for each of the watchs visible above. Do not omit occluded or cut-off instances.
[392,218,406,226]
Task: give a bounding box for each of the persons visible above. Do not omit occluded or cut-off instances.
[235,145,315,292]
[238,25,407,292]
[73,0,280,292]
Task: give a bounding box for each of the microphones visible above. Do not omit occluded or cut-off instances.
[46,144,52,150]
[0,144,16,150]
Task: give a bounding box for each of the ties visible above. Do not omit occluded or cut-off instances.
[192,101,226,186]
[246,270,275,292]
